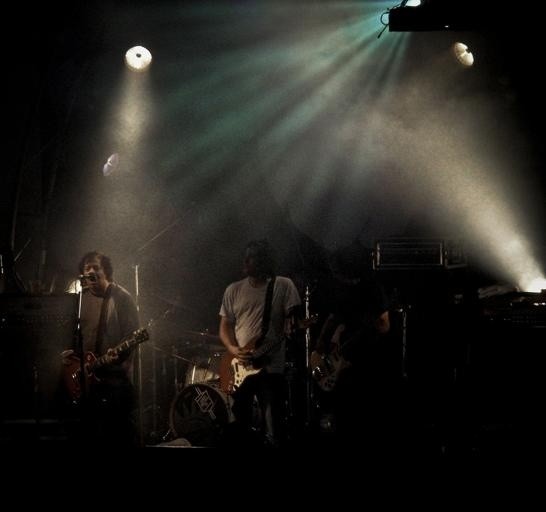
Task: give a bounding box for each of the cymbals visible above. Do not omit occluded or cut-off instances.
[155,295,198,316]
[181,330,221,339]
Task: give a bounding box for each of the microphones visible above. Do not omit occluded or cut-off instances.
[78,274,97,282]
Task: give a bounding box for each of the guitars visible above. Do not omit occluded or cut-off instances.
[218,314,319,396]
[311,311,390,391]
[64,327,149,399]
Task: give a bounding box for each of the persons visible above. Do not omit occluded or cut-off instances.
[61,250,138,373]
[220,238,301,436]
[311,247,390,392]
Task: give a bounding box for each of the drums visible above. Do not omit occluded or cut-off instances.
[184,344,226,386]
[169,381,234,447]
